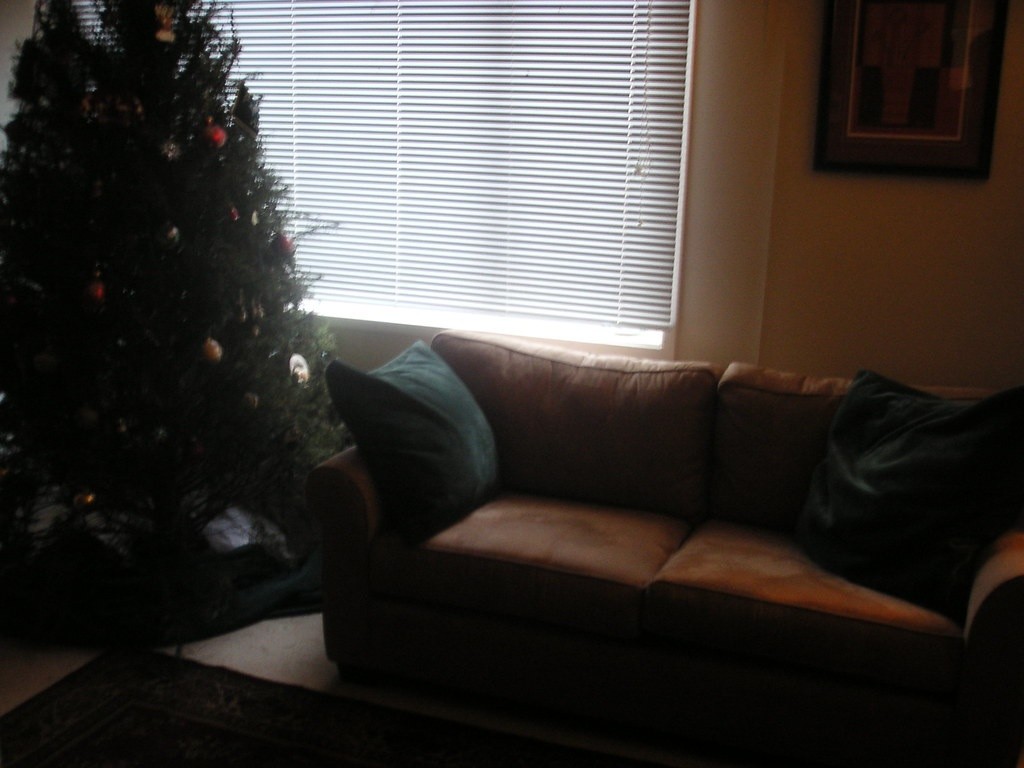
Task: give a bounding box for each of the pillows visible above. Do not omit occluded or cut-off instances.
[325,343,500,549]
[793,371,1024,630]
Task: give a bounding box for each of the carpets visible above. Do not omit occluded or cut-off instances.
[0,647,668,768]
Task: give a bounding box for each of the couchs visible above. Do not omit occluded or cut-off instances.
[305,332,1024,768]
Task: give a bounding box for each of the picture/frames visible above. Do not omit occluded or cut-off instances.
[809,0,1014,182]
